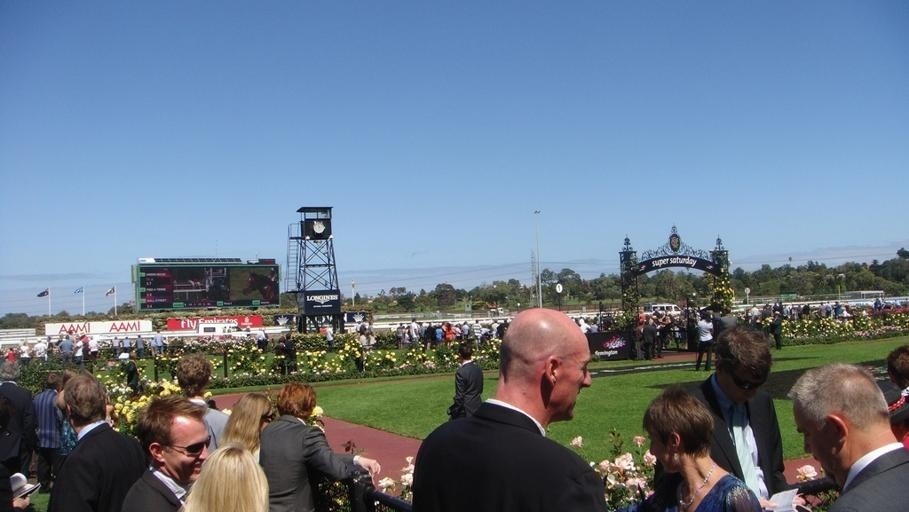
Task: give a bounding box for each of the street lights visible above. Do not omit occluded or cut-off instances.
[533,210,543,308]
[352,279,356,306]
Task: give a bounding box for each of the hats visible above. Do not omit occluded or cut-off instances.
[9,472,41,500]
[877,379,909,422]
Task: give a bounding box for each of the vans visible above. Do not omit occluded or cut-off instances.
[643,302,684,317]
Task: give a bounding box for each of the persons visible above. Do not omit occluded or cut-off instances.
[2,356,381,512]
[441,347,484,421]
[409,309,606,511]
[0,299,909,370]
[642,324,909,512]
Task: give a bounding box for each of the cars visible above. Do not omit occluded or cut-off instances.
[731,296,908,313]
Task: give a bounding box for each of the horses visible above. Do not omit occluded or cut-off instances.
[241,268,277,304]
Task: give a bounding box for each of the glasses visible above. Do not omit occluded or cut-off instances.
[167,436,211,453]
[728,369,761,391]
[263,414,276,420]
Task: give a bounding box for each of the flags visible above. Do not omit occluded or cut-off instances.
[72,286,83,296]
[37,289,49,297]
[106,286,116,297]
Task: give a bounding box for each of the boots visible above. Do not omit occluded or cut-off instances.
[705,361,711,370]
[696,360,701,370]
